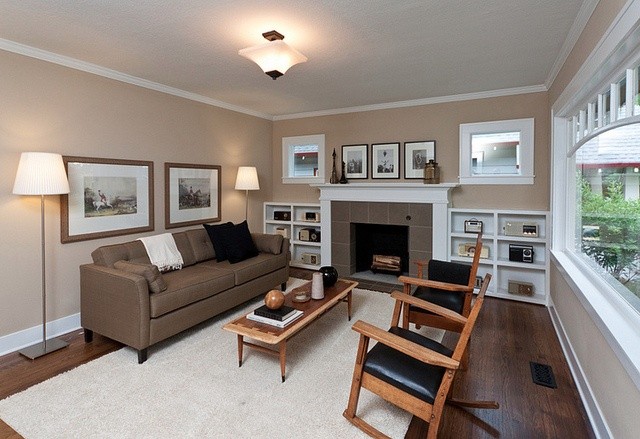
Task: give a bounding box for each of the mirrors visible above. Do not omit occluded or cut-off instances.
[458,118,536,185]
[281,134,325,185]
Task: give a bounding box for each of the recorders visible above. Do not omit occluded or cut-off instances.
[507,279,535,298]
[275,227,291,239]
[502,221,539,238]
[298,227,321,243]
[508,244,535,265]
[300,211,320,223]
[457,243,490,260]
[303,252,321,265]
[474,276,482,289]
[463,219,483,234]
[273,211,291,222]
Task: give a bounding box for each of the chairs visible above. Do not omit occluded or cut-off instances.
[342,273,500,439]
[400,233,484,343]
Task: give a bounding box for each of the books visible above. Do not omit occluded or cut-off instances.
[246,309,304,328]
[254,305,297,322]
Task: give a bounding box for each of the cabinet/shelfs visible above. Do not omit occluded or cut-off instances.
[447,207,552,306]
[262,202,321,271]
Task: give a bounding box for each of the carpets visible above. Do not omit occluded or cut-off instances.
[0,276,446,439]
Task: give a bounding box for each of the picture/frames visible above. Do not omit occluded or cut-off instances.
[60,155,155,245]
[404,140,436,180]
[371,142,400,179]
[341,144,368,180]
[163,161,221,230]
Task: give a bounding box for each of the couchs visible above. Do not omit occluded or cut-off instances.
[80,227,291,364]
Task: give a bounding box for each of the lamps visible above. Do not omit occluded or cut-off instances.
[12,152,71,360]
[238,30,308,80]
[234,166,260,220]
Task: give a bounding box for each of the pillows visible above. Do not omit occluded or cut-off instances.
[200,221,233,262]
[219,220,260,263]
[114,260,170,292]
